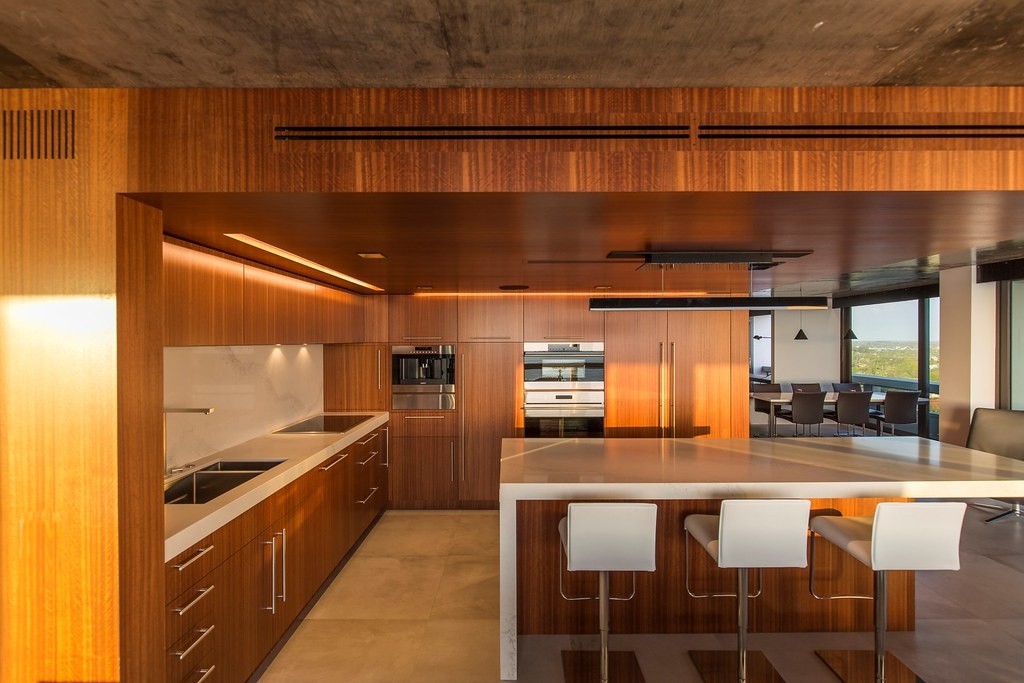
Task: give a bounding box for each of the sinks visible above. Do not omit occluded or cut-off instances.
[164,472,264,505]
[196,460,285,472]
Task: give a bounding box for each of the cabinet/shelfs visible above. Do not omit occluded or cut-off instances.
[319,446,352,586]
[390,293,456,343]
[324,345,387,410]
[525,298,604,340]
[213,466,318,682]
[352,432,373,545]
[459,293,524,509]
[372,420,389,521]
[164,534,215,683]
[605,294,750,438]
[390,409,459,510]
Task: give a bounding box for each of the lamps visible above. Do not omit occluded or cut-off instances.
[844,306,857,340]
[795,307,809,340]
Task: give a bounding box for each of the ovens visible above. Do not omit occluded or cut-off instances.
[391,345,456,410]
[524,342,605,438]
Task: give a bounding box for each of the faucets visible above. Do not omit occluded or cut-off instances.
[163,407,217,477]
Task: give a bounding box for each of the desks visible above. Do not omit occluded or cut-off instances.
[752,393,930,437]
[498,435,1024,682]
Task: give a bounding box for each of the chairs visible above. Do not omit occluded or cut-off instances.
[874,390,921,436]
[823,391,872,436]
[792,382,822,392]
[966,408,1024,525]
[776,392,829,437]
[833,383,862,392]
[753,383,782,439]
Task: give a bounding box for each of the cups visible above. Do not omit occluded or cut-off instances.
[797,389,802,392]
[850,390,856,392]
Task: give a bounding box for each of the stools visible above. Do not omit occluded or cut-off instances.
[811,503,967,683]
[683,500,810,683]
[559,502,656,683]
